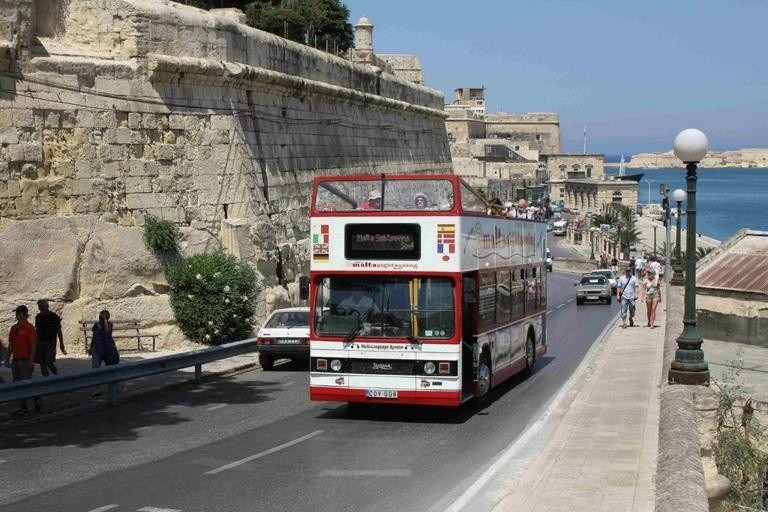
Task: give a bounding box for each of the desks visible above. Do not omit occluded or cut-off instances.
[659,184,671,282]
[652,222,658,256]
[590,226,595,260]
[644,180,650,204]
[671,189,687,286]
[668,128,711,385]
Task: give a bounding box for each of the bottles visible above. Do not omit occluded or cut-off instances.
[624,266,633,271]
[11,305,29,312]
[367,189,382,202]
[413,192,429,203]
[645,268,656,275]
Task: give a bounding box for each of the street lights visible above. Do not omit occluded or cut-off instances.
[617,291,622,302]
[101,345,120,364]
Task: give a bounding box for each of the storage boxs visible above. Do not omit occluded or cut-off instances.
[78,317,160,354]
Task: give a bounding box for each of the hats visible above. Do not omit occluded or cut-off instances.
[257,306,331,370]
[547,248,554,272]
[547,203,568,236]
[570,208,580,214]
[574,269,617,305]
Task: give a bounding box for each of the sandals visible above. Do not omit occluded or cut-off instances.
[300,174,547,407]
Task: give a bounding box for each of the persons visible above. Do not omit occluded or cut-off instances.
[88,309,120,396]
[616,266,640,329]
[598,253,665,288]
[641,268,662,329]
[414,191,548,222]
[334,281,381,321]
[357,190,380,210]
[4,304,42,415]
[34,299,68,377]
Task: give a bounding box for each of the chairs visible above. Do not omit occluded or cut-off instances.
[14,402,46,417]
[622,317,655,330]
[92,384,117,397]
